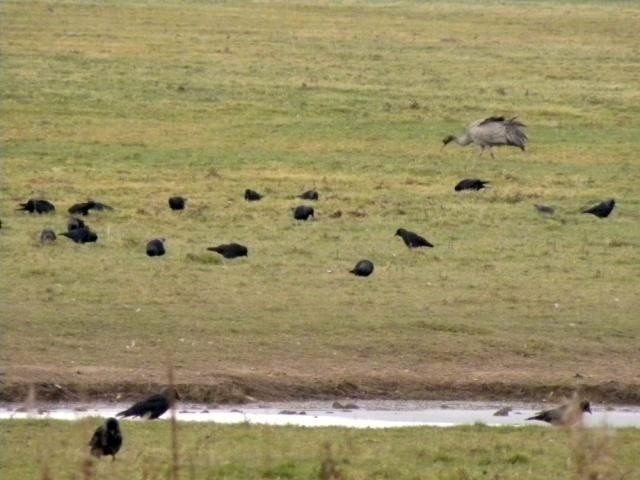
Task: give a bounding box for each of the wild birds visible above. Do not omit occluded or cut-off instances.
[349,260,374,276]
[88,416,122,460]
[114,385,180,422]
[440,114,529,163]
[525,398,591,428]
[581,197,615,221]
[395,227,434,250]
[454,177,492,193]
